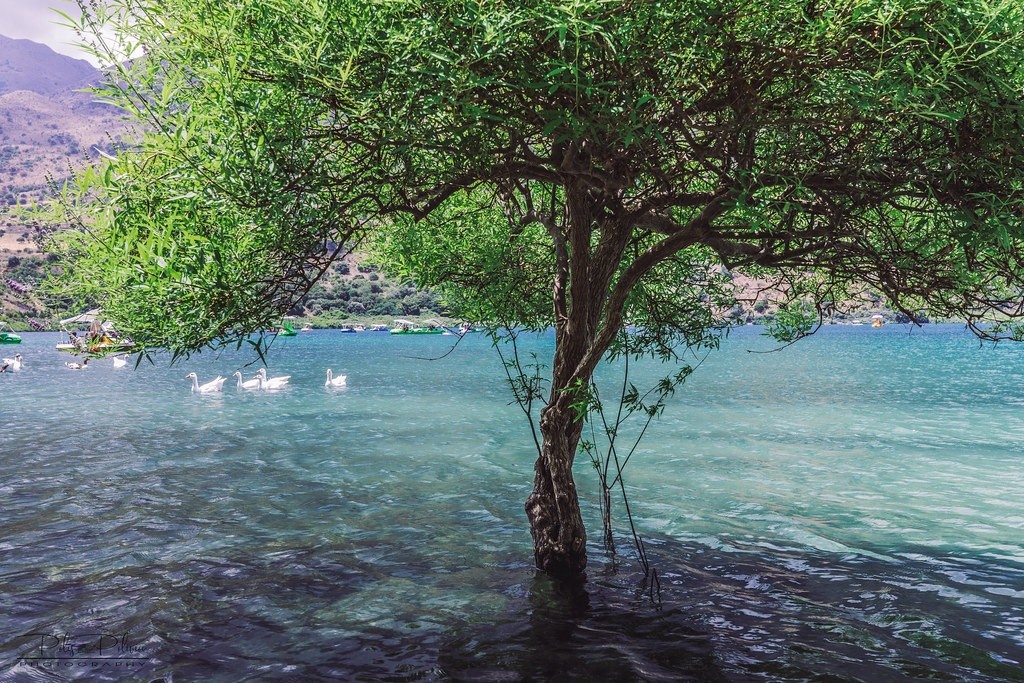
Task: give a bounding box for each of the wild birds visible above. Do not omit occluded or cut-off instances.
[65,358,90,370]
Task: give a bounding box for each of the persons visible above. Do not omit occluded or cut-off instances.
[427,324,438,331]
[346,325,353,328]
[403,324,408,331]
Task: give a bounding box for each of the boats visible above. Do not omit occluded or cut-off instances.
[871,314,885,327]
[278,322,298,336]
[852,320,863,326]
[388,319,450,335]
[370,325,388,331]
[267,317,296,332]
[340,323,366,333]
[458,320,484,333]
[0,321,22,344]
[301,323,313,332]
[54,308,136,353]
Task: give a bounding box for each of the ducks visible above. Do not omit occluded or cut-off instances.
[0,352,25,373]
[113,354,130,368]
[324,369,347,394]
[253,368,291,390]
[233,372,260,392]
[186,373,227,393]
